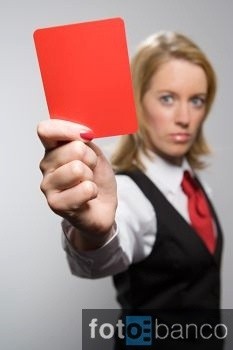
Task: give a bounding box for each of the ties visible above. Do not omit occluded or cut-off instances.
[180,171,216,255]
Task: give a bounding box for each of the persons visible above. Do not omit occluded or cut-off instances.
[37,31,223,350]
